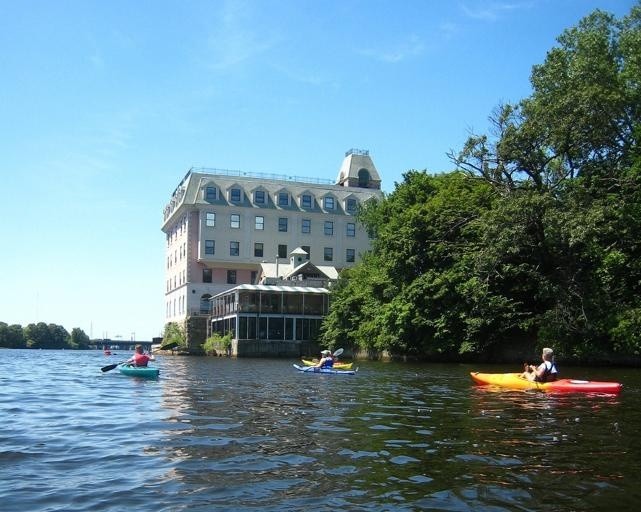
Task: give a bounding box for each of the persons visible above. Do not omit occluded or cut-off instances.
[521,347,560,382]
[312,349,334,362]
[310,350,334,367]
[126,344,156,367]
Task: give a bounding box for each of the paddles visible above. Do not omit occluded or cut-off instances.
[304,348,344,372]
[101,342,179,372]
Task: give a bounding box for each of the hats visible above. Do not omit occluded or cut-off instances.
[320,350,329,355]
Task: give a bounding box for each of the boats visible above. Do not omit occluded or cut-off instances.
[118,361,159,378]
[302,358,354,371]
[469,370,623,396]
[104,350,111,355]
[292,363,356,375]
